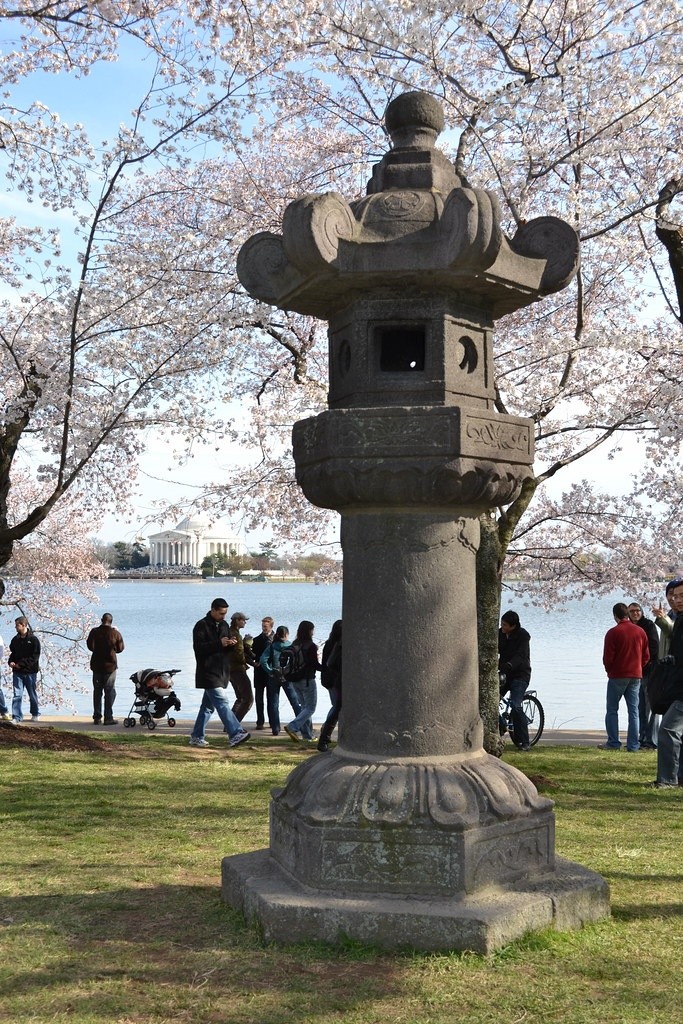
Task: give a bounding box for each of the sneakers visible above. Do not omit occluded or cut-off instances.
[230,732,250,747]
[648,780,678,790]
[188,733,209,747]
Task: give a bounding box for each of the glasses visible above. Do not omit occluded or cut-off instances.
[630,609,641,613]
[673,593,683,599]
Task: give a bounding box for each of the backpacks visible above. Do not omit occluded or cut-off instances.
[279,641,307,682]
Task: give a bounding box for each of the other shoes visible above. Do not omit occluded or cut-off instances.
[256,723,281,735]
[94,718,101,724]
[10,718,23,724]
[625,739,658,753]
[284,726,299,742]
[104,718,118,724]
[303,736,317,743]
[31,716,37,722]
[598,741,620,752]
[522,742,530,751]
[317,741,328,752]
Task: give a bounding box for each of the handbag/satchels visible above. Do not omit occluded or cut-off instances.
[647,655,680,714]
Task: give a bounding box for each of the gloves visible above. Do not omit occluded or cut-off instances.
[500,662,512,675]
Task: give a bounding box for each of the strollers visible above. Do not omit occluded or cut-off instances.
[123,668,183,731]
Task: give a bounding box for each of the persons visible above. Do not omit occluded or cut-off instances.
[251,616,276,731]
[86,612,125,726]
[0,636,9,719]
[316,620,343,751]
[497,610,532,751]
[223,612,254,732]
[188,598,251,747]
[645,580,683,789]
[284,620,322,742]
[628,603,664,751]
[8,616,41,724]
[652,580,678,662]
[597,602,650,753]
[260,625,302,736]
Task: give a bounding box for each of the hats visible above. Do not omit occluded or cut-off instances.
[231,612,249,620]
[243,635,253,648]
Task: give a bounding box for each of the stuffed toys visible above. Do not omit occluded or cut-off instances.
[243,634,260,670]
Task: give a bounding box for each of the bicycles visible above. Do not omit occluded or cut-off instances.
[498,670,545,750]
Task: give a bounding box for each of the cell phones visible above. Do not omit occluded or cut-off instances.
[230,637,236,640]
